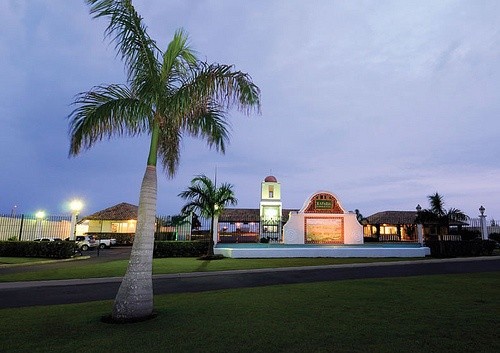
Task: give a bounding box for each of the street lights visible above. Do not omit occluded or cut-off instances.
[416,203,423,244]
[478,206,489,240]
[36,213,44,241]
[70,200,82,255]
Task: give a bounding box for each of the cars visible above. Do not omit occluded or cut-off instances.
[29,234,116,251]
